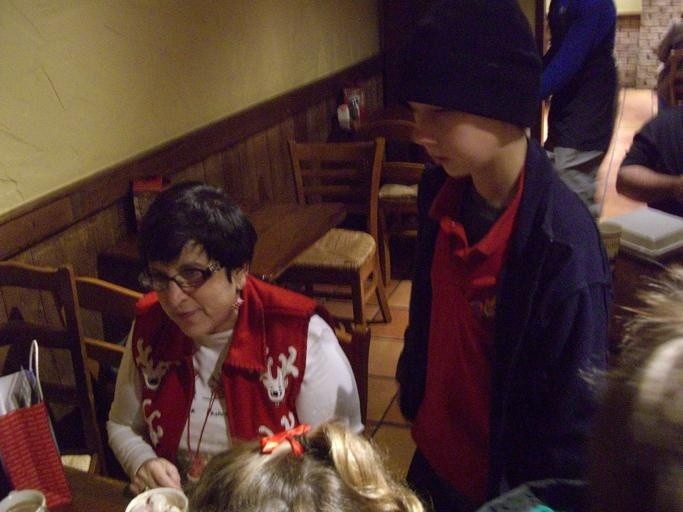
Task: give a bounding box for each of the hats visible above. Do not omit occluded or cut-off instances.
[396,0,543,128]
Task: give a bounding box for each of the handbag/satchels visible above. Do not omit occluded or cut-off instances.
[0,338,73,509]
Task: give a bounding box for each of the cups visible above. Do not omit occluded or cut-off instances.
[0,489,49,512]
[596,222,622,278]
[125,487,189,512]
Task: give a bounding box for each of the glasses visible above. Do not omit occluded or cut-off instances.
[138,260,226,291]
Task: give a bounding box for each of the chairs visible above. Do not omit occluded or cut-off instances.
[289,106,435,435]
[0,260,144,475]
[654,48,682,106]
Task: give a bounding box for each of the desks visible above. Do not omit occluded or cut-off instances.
[100,203,345,283]
